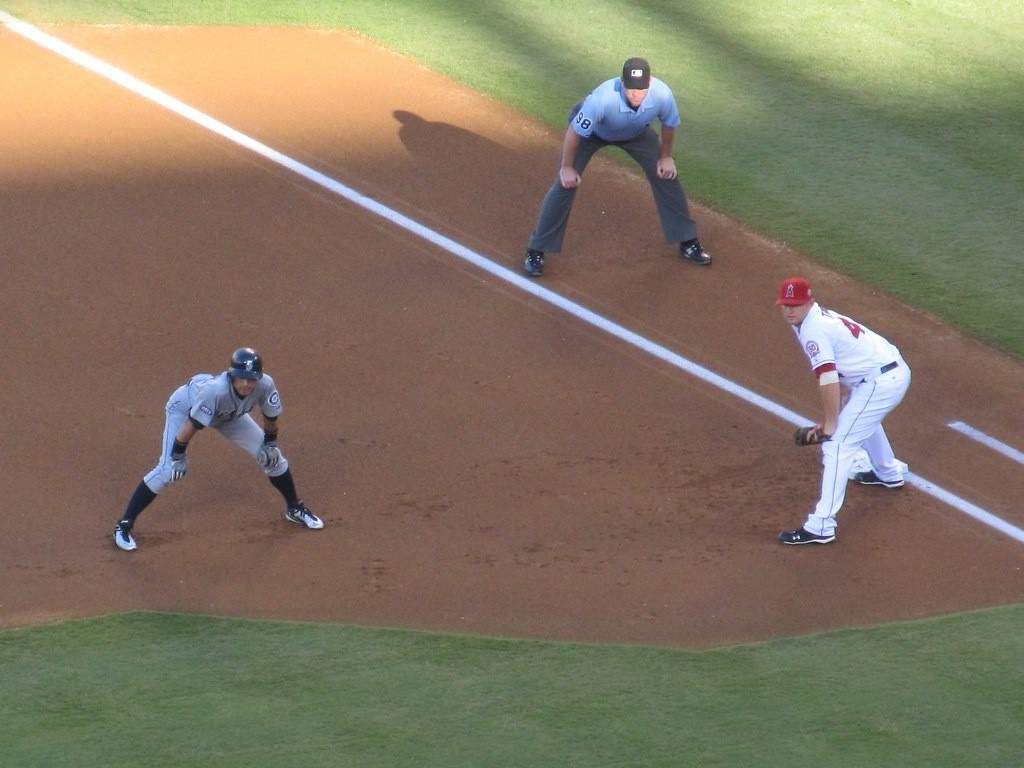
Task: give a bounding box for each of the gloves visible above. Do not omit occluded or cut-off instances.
[257,429,280,469]
[793,425,828,445]
[170,436,190,481]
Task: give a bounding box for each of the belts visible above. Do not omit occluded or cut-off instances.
[860,361,898,384]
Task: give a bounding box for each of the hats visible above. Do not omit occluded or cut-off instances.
[624,58,650,89]
[775,278,812,305]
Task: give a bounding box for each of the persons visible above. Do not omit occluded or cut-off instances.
[524,58,711,277]
[776,279,910,543]
[112,348,324,549]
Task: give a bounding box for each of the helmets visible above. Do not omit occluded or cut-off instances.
[227,347,263,380]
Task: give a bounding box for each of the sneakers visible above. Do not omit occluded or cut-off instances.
[679,238,711,264]
[285,500,324,530]
[854,470,905,489]
[111,520,137,551]
[524,249,546,276]
[778,528,835,544]
